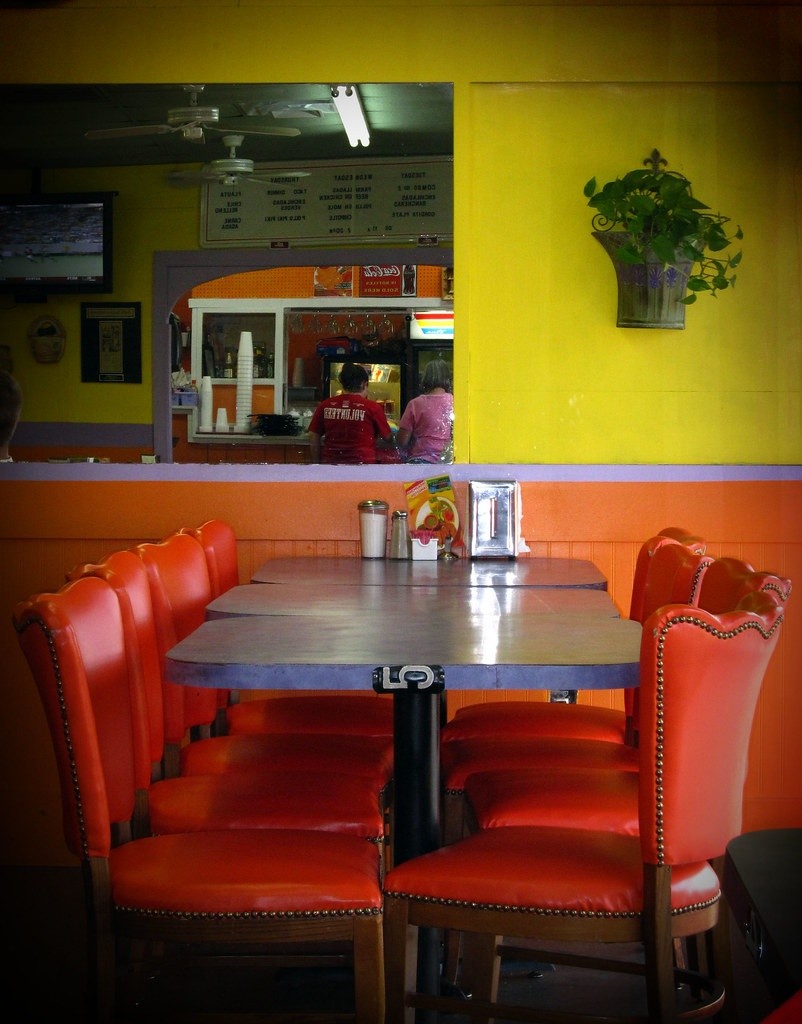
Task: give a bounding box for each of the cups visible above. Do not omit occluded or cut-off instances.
[358,500,389,559]
[236,332,253,425]
[201,376,214,432]
[216,408,229,432]
[293,357,305,386]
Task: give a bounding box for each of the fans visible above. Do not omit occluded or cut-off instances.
[83,83,301,142]
[160,134,312,187]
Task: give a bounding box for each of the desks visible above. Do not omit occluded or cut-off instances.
[204,583,621,624]
[249,556,608,590]
[164,617,645,1024]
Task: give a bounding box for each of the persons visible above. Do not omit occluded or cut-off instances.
[24,245,54,264]
[397,360,454,464]
[0,370,23,463]
[307,365,394,465]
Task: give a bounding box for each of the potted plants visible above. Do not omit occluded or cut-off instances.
[582,167,743,331]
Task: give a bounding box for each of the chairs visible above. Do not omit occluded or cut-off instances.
[12,520,792,1023]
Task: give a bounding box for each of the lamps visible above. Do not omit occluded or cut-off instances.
[330,84,371,148]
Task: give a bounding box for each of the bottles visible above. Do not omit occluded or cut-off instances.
[223,353,234,378]
[253,342,274,378]
[389,510,412,559]
